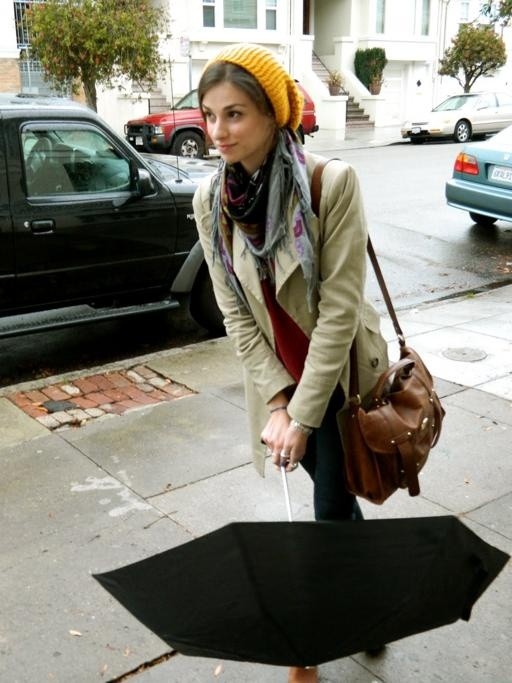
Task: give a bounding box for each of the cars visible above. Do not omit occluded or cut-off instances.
[445,120,512,227]
[401,92,512,143]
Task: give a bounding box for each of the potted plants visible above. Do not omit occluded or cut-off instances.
[355,46,387,95]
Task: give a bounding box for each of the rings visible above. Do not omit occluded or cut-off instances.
[289,462,298,469]
[271,452,279,456]
[279,450,290,457]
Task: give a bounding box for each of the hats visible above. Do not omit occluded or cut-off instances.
[201,44,304,133]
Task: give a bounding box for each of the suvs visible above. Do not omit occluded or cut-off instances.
[125,66,319,159]
[0,92,223,381]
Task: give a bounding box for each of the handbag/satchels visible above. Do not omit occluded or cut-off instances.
[343,345,447,506]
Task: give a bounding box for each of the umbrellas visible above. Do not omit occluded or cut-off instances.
[87,463,512,670]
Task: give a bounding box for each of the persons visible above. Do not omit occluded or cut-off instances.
[190,43,392,682]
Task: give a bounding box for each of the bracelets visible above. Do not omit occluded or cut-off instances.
[269,405,287,412]
[291,419,313,435]
